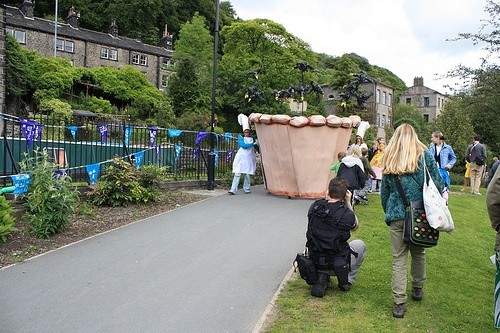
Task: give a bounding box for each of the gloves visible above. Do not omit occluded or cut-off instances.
[254,140,258,145]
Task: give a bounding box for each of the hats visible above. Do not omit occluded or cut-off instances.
[238,113,251,132]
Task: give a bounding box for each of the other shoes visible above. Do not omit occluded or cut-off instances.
[245,190,250,193]
[392,302,408,318]
[356,195,368,204]
[311,272,327,298]
[338,282,351,292]
[227,190,235,195]
[470,190,474,194]
[411,287,422,300]
[460,188,466,192]
[473,191,482,196]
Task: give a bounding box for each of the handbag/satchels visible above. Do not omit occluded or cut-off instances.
[292,253,318,285]
[421,151,454,234]
[325,237,358,282]
[439,168,451,192]
[476,156,485,166]
[467,154,472,163]
[403,201,440,248]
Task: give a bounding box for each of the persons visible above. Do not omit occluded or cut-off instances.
[429,130,456,205]
[228,129,259,195]
[462,135,487,195]
[331,135,387,206]
[381,123,442,318]
[306,178,366,290]
[485,157,500,329]
